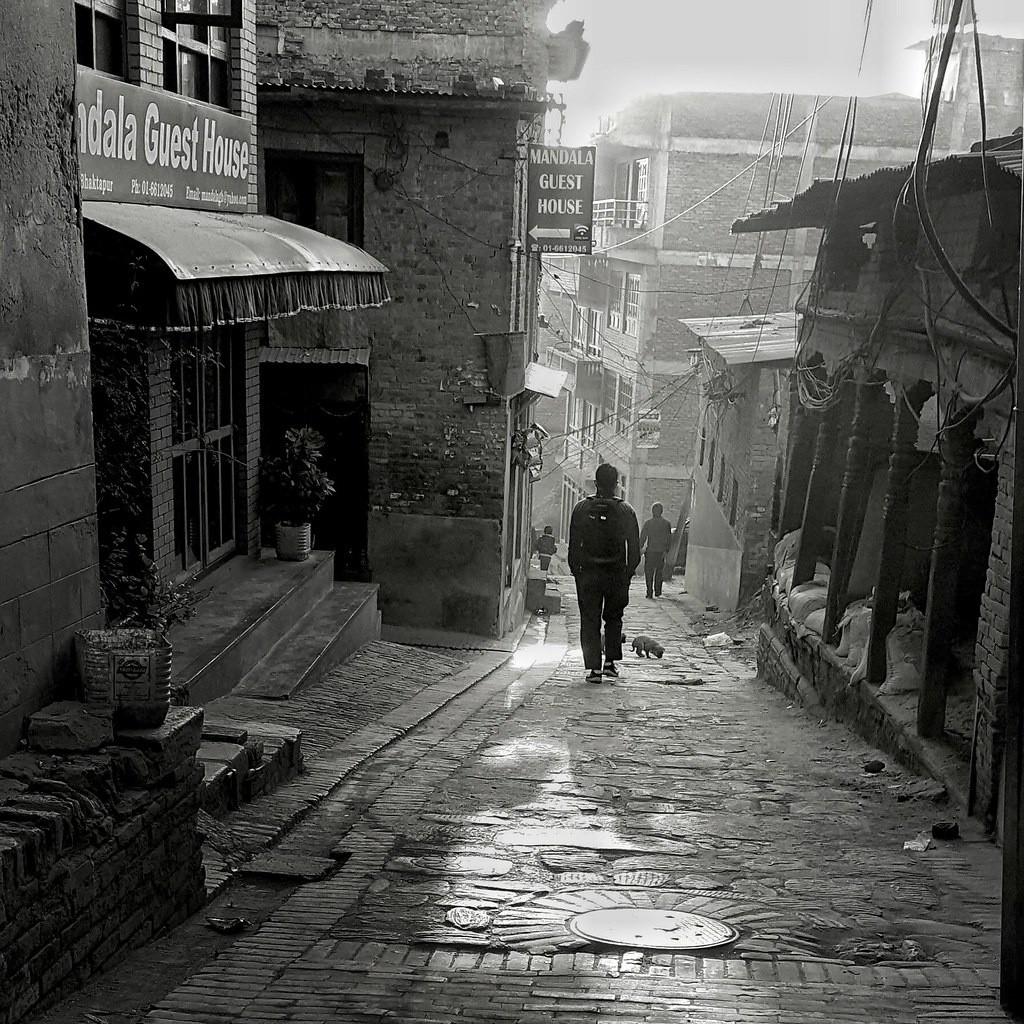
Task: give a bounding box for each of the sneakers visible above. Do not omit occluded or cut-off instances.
[603,660,618,677]
[586,670,603,684]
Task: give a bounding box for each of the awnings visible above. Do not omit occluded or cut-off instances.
[75,199,391,335]
[523,360,568,399]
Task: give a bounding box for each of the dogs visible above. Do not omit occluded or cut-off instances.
[601,633,627,653]
[629,636,665,659]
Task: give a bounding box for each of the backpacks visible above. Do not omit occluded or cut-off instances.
[580,497,624,555]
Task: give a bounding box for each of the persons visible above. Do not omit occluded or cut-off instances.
[567,463,644,683]
[534,525,557,570]
[639,502,671,600]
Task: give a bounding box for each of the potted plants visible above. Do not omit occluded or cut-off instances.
[270,425,336,562]
